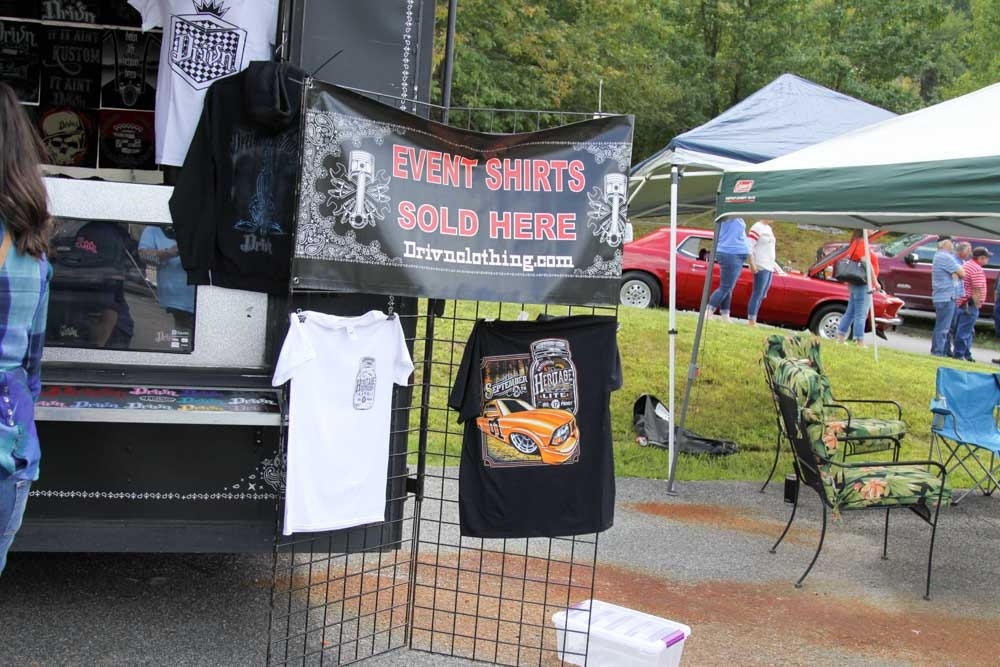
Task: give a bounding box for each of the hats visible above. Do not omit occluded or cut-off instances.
[973,246,994,257]
[935,235,951,242]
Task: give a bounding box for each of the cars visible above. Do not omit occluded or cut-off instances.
[809,227,1000,339]
[618,225,908,343]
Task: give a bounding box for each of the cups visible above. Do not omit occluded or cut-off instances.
[783,472,797,506]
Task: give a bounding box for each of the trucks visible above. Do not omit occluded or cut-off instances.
[2,0,467,580]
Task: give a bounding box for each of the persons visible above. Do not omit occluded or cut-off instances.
[697,248,710,260]
[138,224,200,352]
[74,220,136,349]
[0,78,56,576]
[991,272,1000,363]
[747,217,777,325]
[706,216,748,323]
[930,234,995,362]
[835,228,882,350]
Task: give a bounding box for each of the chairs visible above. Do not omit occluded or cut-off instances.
[926,365,1000,508]
[769,357,952,602]
[754,332,907,562]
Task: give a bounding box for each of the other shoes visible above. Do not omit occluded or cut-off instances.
[991,358,1000,365]
[958,357,965,361]
[966,357,976,362]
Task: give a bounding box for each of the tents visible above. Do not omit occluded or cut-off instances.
[624,69,903,480]
[666,83,1000,499]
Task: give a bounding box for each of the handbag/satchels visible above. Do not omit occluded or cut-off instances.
[832,239,868,285]
